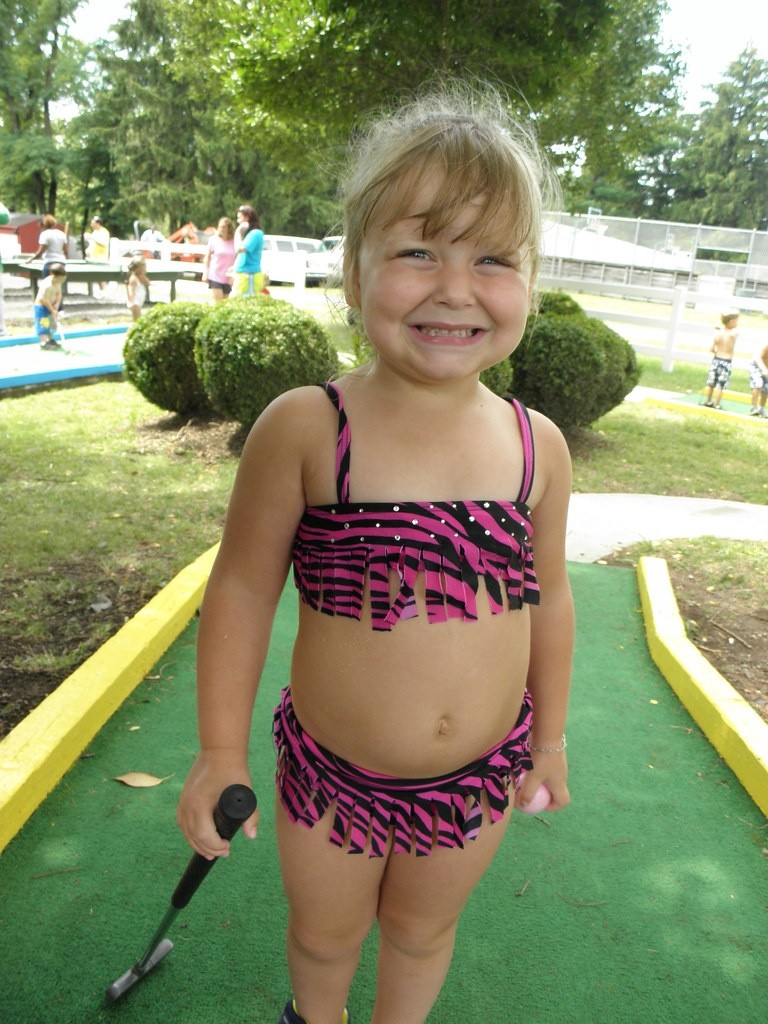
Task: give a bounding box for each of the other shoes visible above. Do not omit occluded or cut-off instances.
[702,401,721,410]
[41,339,61,352]
[750,406,768,419]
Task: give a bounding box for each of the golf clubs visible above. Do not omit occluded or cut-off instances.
[103,783,259,1004]
[54,314,71,356]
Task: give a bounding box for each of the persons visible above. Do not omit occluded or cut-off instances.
[26,215,70,278]
[127,256,150,321]
[141,226,165,253]
[85,216,110,300]
[748,345,768,417]
[34,263,67,350]
[202,217,237,300]
[178,114,571,1023]
[699,308,738,410]
[226,205,269,301]
